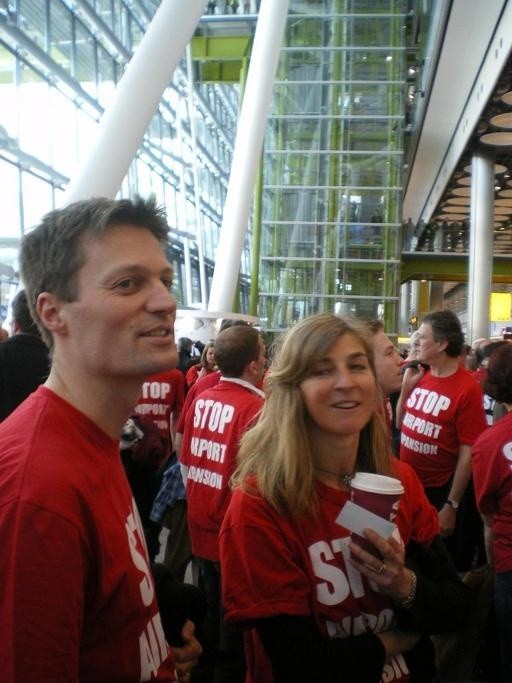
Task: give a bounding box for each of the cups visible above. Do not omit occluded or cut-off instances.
[347,472,405,566]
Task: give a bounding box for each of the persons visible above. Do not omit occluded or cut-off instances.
[123,319,274,683]
[216,314,472,681]
[1,290,51,422]
[360,309,511,683]
[0,192,203,682]
[371,208,470,254]
